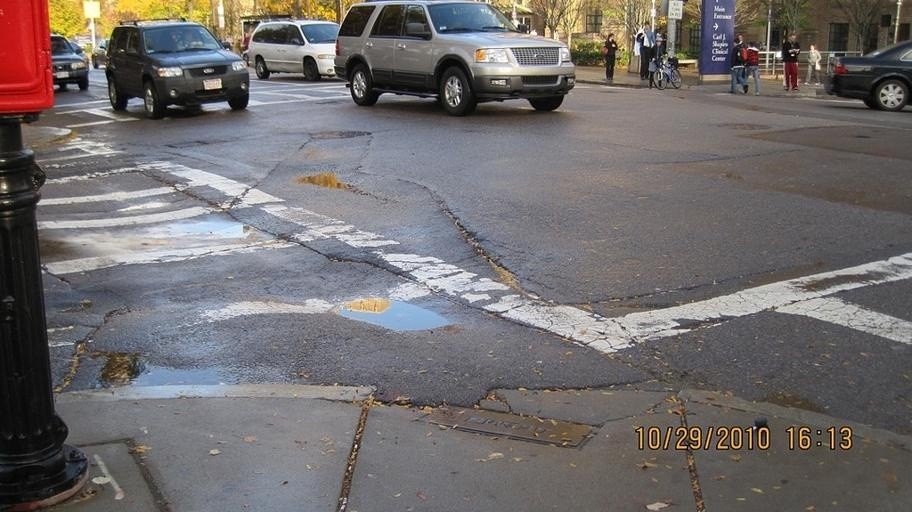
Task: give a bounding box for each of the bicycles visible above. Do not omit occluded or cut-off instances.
[649,55,682,90]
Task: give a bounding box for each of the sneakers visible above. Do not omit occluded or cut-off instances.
[804,82,821,86]
[729,85,748,93]
[753,92,759,96]
[784,87,798,91]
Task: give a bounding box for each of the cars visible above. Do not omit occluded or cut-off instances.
[92,39,109,68]
[242,38,251,62]
[826,37,911,110]
[69,41,90,72]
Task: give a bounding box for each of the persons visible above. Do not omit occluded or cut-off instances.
[637,23,655,80]
[648,37,664,90]
[804,43,821,86]
[783,32,800,91]
[729,34,760,96]
[177,30,204,49]
[605,33,618,80]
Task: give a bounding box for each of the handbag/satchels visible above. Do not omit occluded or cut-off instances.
[648,62,656,72]
[601,48,608,56]
[815,61,821,71]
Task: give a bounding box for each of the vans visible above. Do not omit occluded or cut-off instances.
[246,21,343,81]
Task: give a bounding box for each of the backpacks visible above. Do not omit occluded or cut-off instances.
[736,45,748,62]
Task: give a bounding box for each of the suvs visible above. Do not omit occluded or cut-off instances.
[334,1,575,116]
[50,34,89,92]
[105,17,249,120]
